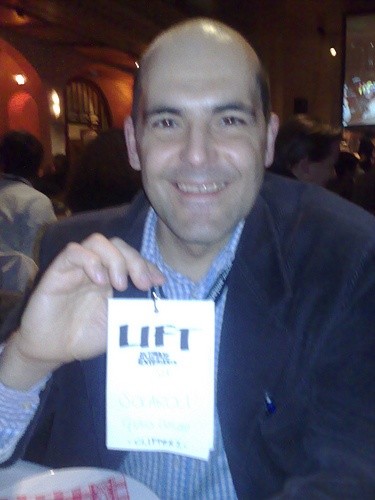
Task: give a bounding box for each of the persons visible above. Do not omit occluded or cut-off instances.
[39,97,375,220]
[1,129,59,343]
[0,17,375,499]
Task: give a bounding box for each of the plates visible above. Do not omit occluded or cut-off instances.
[0,466,162,500]
